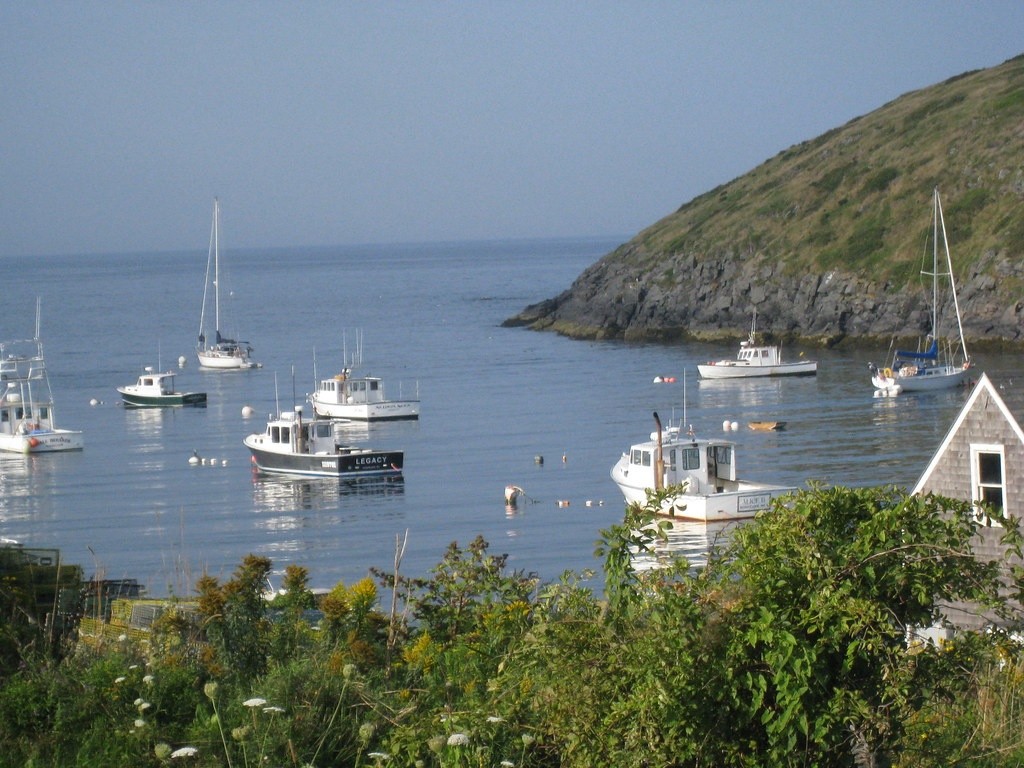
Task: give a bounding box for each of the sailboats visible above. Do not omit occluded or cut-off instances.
[195,196,263,370]
[867,185,976,399]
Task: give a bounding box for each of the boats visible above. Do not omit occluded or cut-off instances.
[697,304,818,379]
[242,364,404,477]
[0,295,84,453]
[116,340,207,408]
[609,368,799,523]
[305,327,421,423]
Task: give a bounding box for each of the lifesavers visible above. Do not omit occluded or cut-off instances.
[883,368,892,378]
